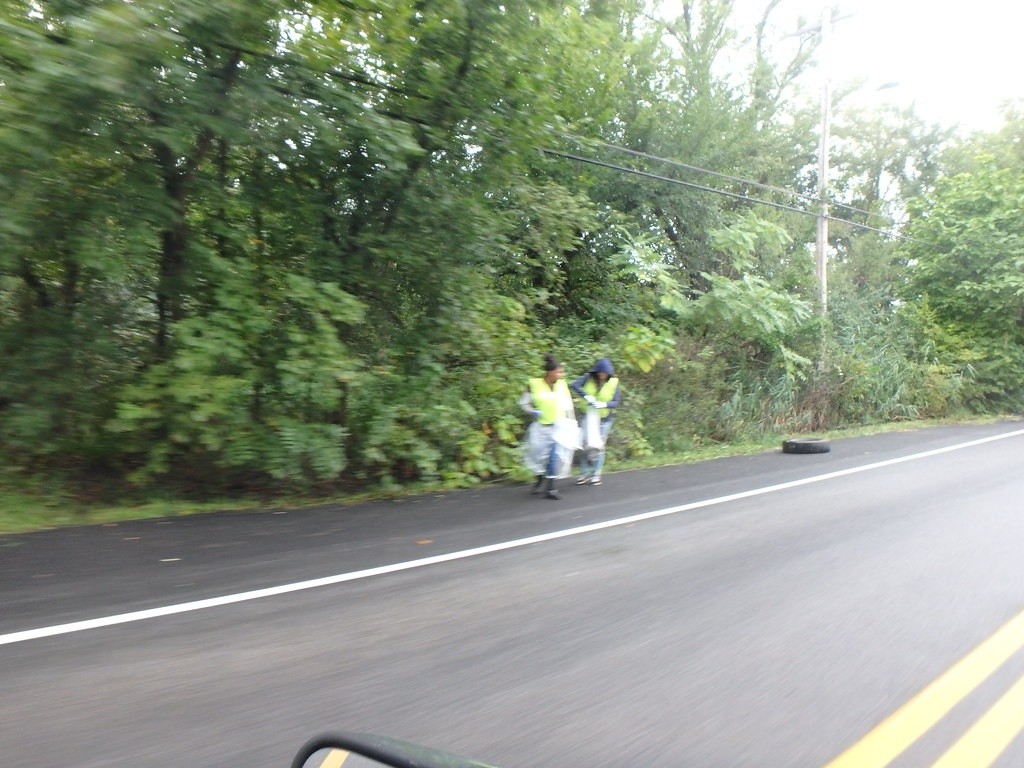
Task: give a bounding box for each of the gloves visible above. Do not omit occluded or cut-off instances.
[530,409,543,419]
[593,401,608,409]
[584,395,596,406]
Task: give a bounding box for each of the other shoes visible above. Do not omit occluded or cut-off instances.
[542,489,563,500]
[590,477,604,485]
[576,474,595,485]
[532,478,554,495]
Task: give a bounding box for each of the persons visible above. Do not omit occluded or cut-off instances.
[518,354,577,500]
[568,358,622,485]
